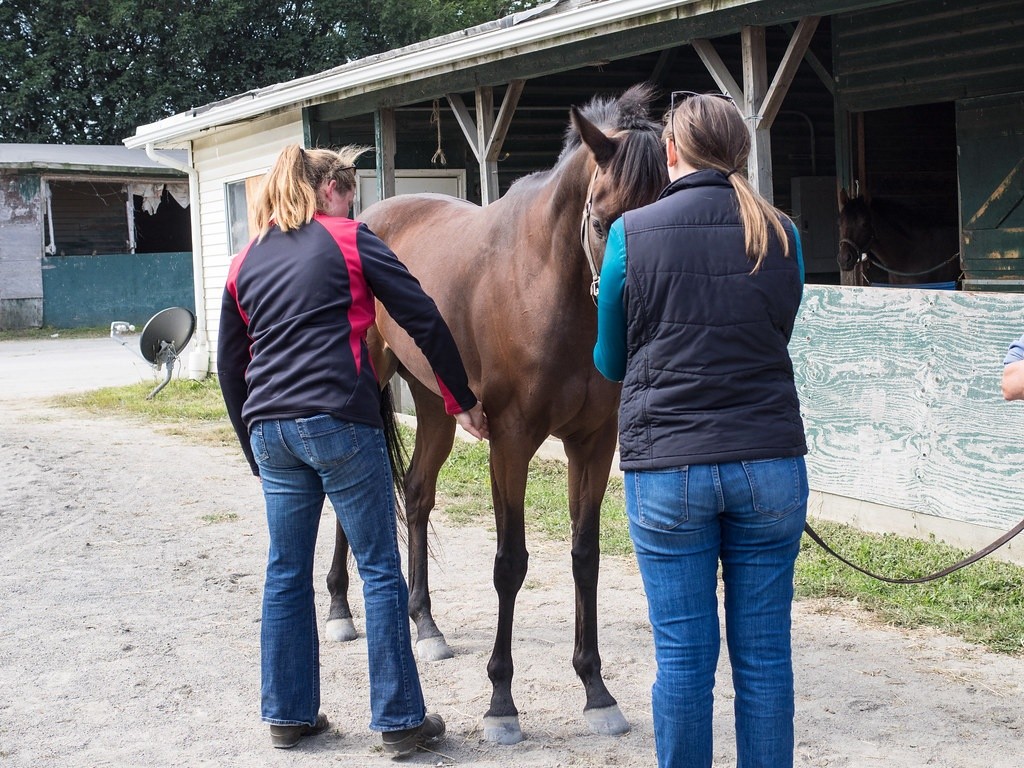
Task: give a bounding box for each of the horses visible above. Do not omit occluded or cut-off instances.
[836,170,960,285]
[326,81,672,747]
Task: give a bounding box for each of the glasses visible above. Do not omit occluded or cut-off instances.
[671,91,733,145]
[325,163,356,186]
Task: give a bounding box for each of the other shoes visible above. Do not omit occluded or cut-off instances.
[382,712,446,754]
[269,711,330,748]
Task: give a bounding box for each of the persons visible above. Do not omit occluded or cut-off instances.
[593,92,811,768]
[1001,334,1024,401]
[217,146,487,758]
